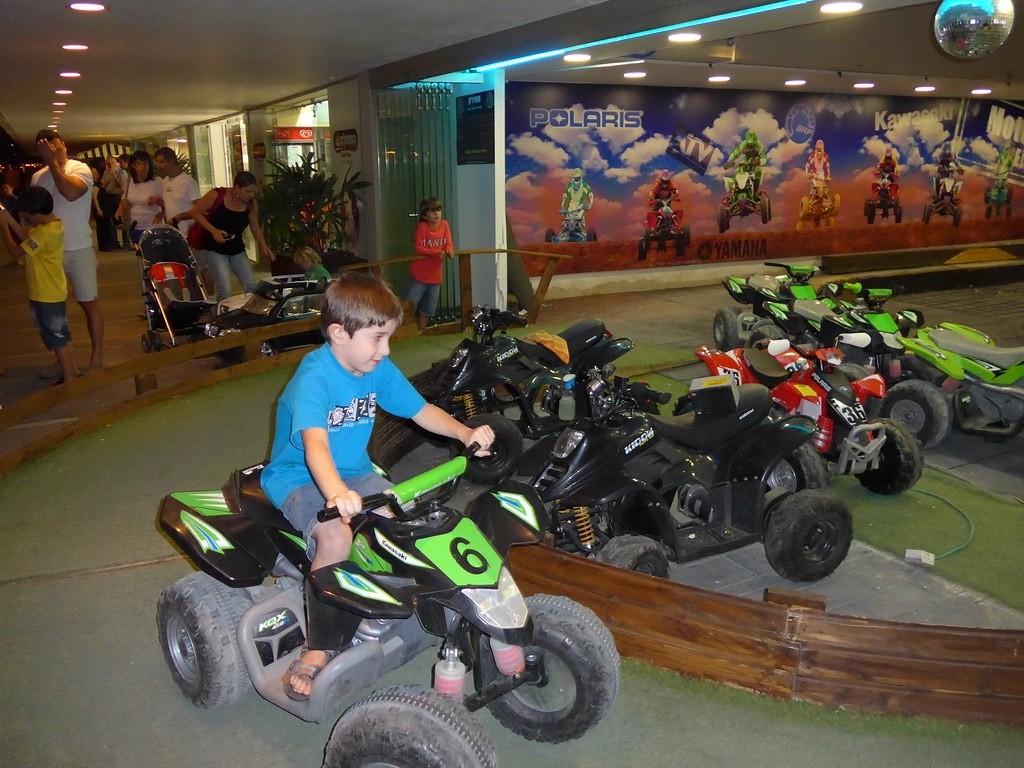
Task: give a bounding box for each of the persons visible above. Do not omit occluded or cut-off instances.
[648,168,679,212]
[992,140,1014,194]
[257,269,498,701]
[403,197,455,329]
[723,128,767,202]
[560,167,594,234]
[933,141,966,201]
[91,145,278,307]
[875,145,900,204]
[805,140,830,196]
[0,129,104,380]
[293,245,334,295]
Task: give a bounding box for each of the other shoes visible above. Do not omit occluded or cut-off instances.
[39,362,63,378]
[122,242,136,250]
[76,365,97,377]
[137,307,149,318]
[6,260,22,269]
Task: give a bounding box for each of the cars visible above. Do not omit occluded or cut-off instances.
[203,273,339,363]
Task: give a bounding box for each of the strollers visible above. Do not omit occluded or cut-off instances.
[128,219,218,353]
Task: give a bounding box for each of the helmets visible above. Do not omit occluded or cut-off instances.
[661,169,671,188]
[883,146,893,169]
[745,129,758,153]
[1003,140,1011,156]
[815,139,824,160]
[942,141,952,161]
[570,168,583,192]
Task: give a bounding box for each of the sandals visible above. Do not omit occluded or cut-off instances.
[282,645,340,702]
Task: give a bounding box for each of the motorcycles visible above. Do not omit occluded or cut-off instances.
[863,166,903,224]
[544,204,597,242]
[983,168,1014,219]
[715,158,772,233]
[921,164,966,228]
[796,174,840,231]
[154,431,622,768]
[423,262,1024,582]
[638,193,691,262]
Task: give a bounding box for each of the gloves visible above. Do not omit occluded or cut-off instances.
[723,162,731,169]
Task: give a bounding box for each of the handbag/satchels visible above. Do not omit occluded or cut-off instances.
[187,186,226,250]
[120,177,131,230]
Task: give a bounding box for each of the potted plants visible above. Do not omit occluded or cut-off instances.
[254,153,375,276]
